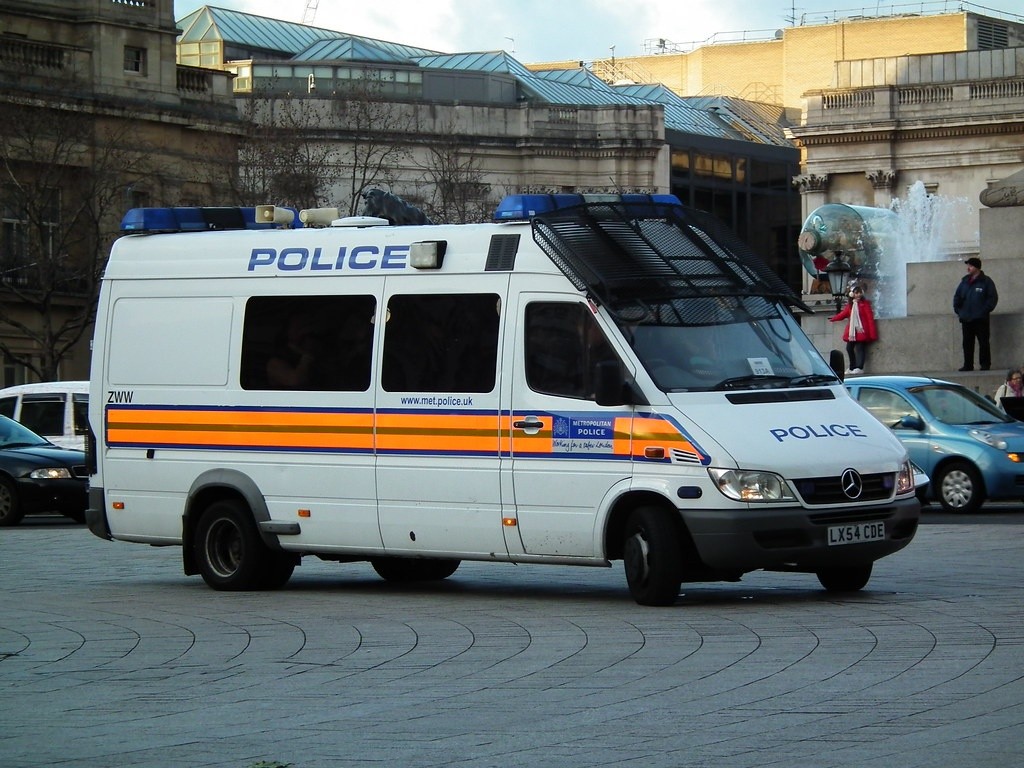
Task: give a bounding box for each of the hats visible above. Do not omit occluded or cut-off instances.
[965,258,981,269]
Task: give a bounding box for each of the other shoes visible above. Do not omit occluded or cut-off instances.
[959,366,974,372]
[980,367,990,372]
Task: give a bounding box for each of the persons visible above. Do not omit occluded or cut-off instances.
[827,286,878,374]
[953,258,998,371]
[561,304,604,351]
[994,370,1024,413]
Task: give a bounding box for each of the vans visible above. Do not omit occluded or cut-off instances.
[0,381,90,452]
[88,192,922,610]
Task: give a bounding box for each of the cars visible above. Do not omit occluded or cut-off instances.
[837,375,1024,516]
[0,414,89,528]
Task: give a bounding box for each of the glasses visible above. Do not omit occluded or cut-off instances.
[1008,377,1022,381]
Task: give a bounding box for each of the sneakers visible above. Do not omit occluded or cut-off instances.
[844,367,853,374]
[850,367,864,374]
